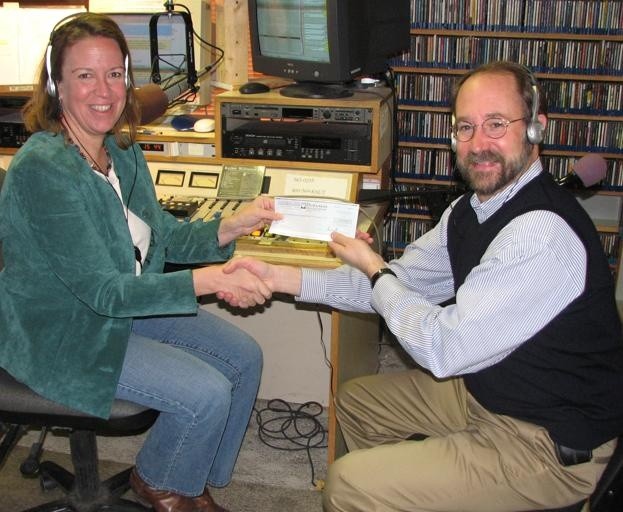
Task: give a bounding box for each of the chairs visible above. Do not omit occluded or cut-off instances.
[585,437,622,511]
[0,370,161,512]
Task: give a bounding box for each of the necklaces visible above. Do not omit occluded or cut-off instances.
[66,136,111,177]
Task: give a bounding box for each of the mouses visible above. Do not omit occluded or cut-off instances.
[193,118,215,133]
[239,83,270,95]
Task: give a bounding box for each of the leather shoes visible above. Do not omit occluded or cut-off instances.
[129,468,227,512]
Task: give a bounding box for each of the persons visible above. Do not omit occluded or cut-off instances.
[215,58,620,512]
[1,12,287,511]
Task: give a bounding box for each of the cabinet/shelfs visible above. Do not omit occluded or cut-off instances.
[386,0,622,274]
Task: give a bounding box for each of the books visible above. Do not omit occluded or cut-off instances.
[379,0,623,285]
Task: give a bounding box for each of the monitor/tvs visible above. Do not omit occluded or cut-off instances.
[89,0,211,107]
[0,0,89,96]
[248,0,410,99]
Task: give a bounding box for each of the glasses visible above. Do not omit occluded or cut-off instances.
[450,116,526,143]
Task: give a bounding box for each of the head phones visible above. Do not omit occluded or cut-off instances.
[46,12,131,97]
[449,64,547,153]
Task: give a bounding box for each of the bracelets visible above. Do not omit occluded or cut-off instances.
[369,269,398,288]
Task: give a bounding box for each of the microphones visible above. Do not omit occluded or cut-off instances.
[129,75,189,125]
[555,154,607,197]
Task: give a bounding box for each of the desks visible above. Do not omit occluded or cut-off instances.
[155,197,390,480]
